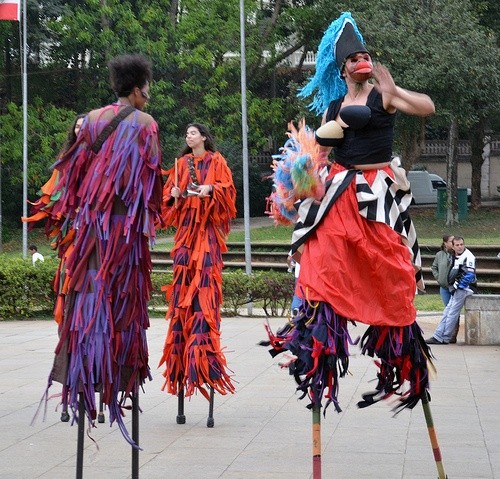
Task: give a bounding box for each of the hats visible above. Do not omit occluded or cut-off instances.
[297,13,372,117]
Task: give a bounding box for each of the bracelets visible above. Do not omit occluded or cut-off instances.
[208,184,212,196]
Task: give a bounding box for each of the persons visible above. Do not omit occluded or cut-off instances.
[257,13,437,417]
[432,234,459,343]
[160,123,240,400]
[22,53,164,451]
[425,237,476,345]
[28,245,44,268]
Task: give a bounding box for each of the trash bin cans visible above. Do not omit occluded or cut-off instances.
[437,187,468,223]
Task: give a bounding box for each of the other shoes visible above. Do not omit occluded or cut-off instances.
[425,337,448,344]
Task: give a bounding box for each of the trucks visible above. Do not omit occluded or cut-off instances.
[406,169,447,206]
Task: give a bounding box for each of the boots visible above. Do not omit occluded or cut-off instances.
[449,325,460,343]
